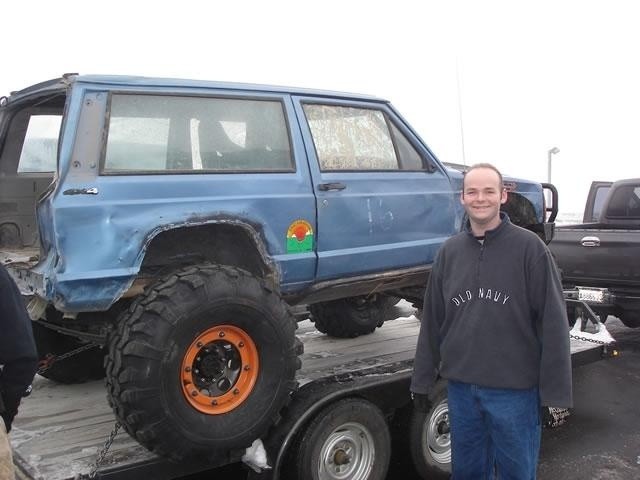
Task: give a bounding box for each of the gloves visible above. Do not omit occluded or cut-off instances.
[410,391,434,414]
[543,407,570,429]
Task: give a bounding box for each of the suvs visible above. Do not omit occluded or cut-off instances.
[0,71,558,468]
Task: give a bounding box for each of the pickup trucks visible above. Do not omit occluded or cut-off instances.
[545,178,640,328]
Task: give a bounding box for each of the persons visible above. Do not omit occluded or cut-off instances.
[409,164,572,480]
[0,261,39,480]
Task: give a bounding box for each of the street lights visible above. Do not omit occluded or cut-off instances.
[549,147,559,208]
